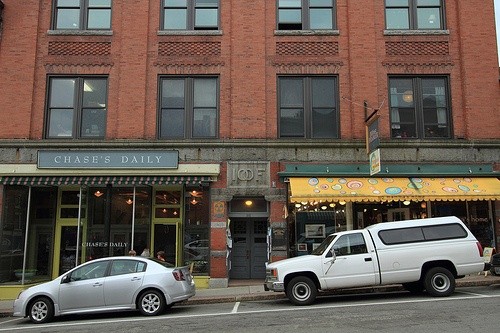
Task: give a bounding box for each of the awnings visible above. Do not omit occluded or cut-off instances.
[287,176,500,205]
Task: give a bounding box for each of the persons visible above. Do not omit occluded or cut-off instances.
[129,250,136,256]
[157,251,167,262]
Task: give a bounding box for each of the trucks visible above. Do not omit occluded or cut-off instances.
[264,216,492,306]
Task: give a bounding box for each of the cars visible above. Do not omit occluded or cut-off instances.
[490,253,500,276]
[12,256,196,323]
